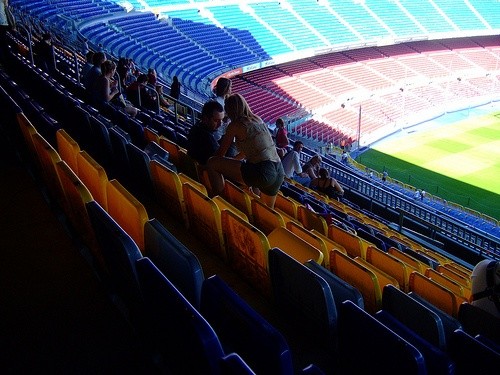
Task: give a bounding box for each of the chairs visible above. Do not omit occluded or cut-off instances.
[0,0,500,375]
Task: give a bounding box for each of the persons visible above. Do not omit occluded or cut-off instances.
[171,76,182,100]
[81,50,137,117]
[281,141,311,188]
[211,78,236,157]
[115,57,174,111]
[36,33,53,73]
[342,151,348,165]
[340,139,346,150]
[421,189,425,200]
[470,259,500,318]
[302,154,322,179]
[382,170,389,182]
[369,171,374,179]
[413,189,420,200]
[270,118,290,155]
[207,94,285,210]
[310,168,345,202]
[190,100,224,166]
